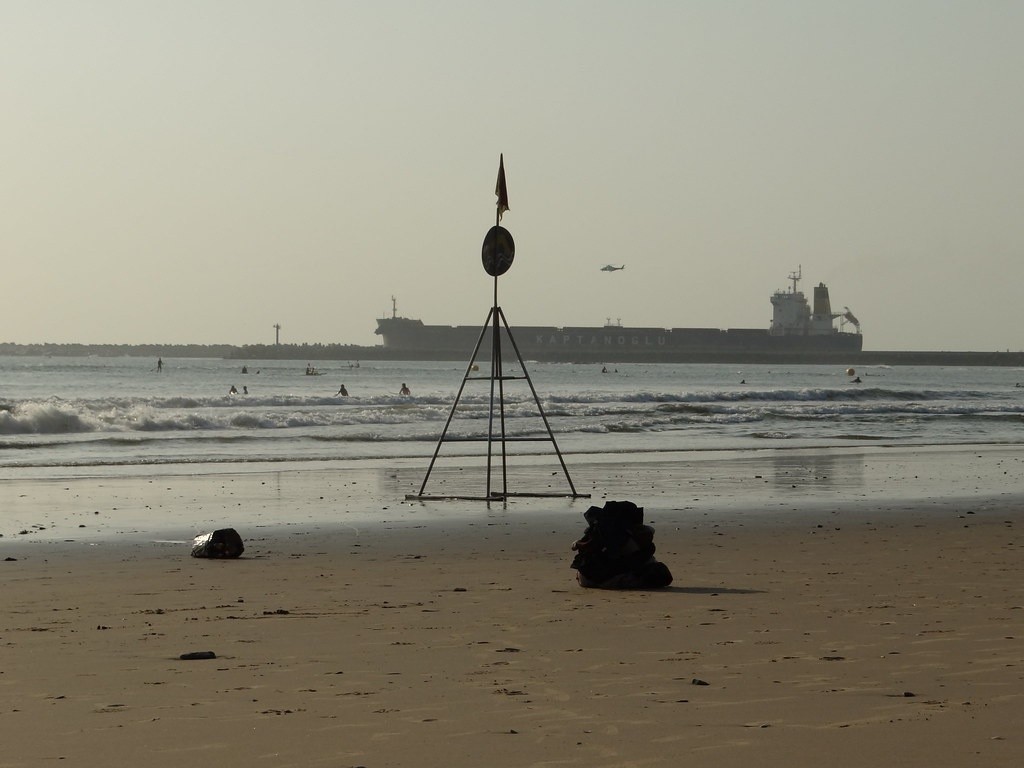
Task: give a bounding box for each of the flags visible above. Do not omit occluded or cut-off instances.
[495,154,511,221]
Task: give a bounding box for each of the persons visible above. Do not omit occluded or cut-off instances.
[337,384,348,396]
[244,386,248,394]
[229,385,238,394]
[157,357,163,372]
[399,383,410,395]
[306,363,315,374]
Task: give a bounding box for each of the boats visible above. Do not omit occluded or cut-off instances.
[304,371,324,375]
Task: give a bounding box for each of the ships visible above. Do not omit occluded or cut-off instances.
[371,266,863,365]
